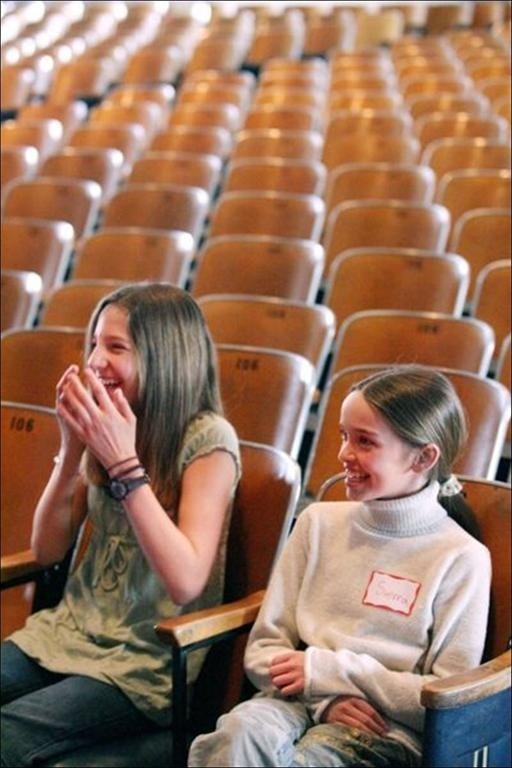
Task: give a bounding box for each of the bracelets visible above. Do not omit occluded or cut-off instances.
[110,464,144,479]
[104,455,140,472]
[53,454,83,479]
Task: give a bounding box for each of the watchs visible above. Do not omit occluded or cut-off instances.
[108,474,151,502]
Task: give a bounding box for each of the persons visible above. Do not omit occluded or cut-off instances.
[187,366,494,766]
[0,281,244,767]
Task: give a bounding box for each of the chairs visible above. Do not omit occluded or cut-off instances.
[315,465,511,766]
[495,333,511,397]
[207,344,318,473]
[470,260,510,375]
[76,227,199,297]
[451,206,510,315]
[324,247,468,353]
[2,325,89,414]
[322,198,451,291]
[195,291,339,403]
[1,2,511,232]
[2,216,74,324]
[189,232,328,305]
[324,306,492,388]
[2,401,66,640]
[302,363,511,526]
[56,441,304,768]
